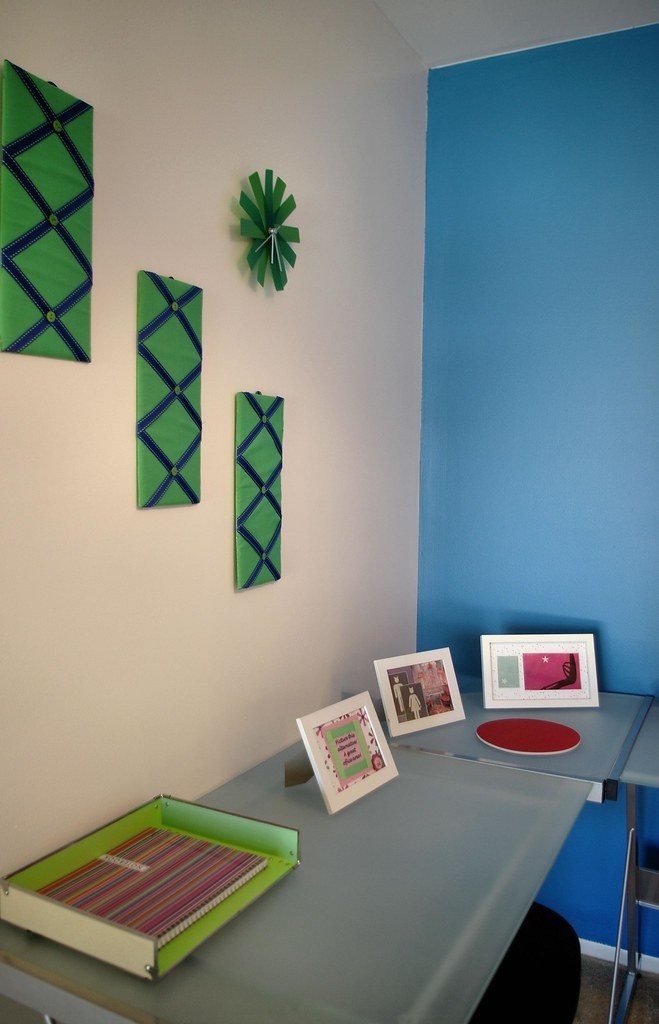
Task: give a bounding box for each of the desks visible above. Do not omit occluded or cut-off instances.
[0,672,659,1024]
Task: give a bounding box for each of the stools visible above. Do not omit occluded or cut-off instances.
[468,902,582,1024]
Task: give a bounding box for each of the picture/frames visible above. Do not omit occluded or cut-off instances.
[374,647,466,738]
[480,634,599,709]
[283,689,399,815]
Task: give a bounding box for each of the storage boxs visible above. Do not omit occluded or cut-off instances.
[0,793,300,984]
